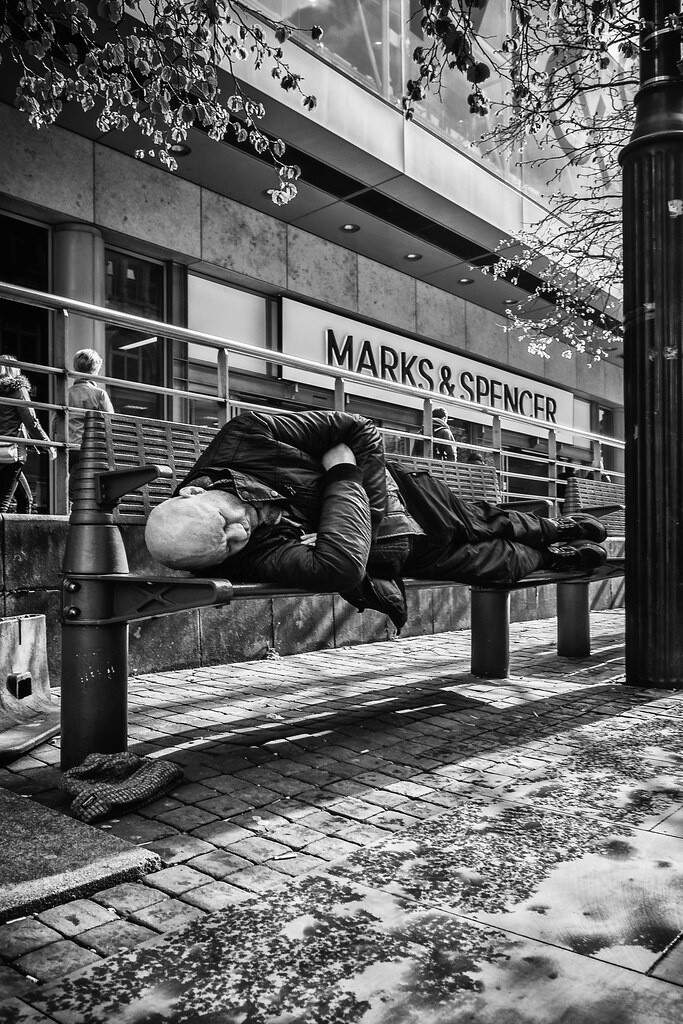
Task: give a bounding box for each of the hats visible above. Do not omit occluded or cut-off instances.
[60,753,188,825]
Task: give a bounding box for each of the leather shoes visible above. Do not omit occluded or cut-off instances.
[544,541,607,571]
[541,512,607,544]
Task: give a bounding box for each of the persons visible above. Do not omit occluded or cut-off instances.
[145,409,609,629]
[49,348,116,470]
[0,355,56,514]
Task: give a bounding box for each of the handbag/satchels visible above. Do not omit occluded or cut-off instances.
[0,442,18,465]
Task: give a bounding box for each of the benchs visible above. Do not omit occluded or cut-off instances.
[554,477,625,657]
[58,411,599,771]
